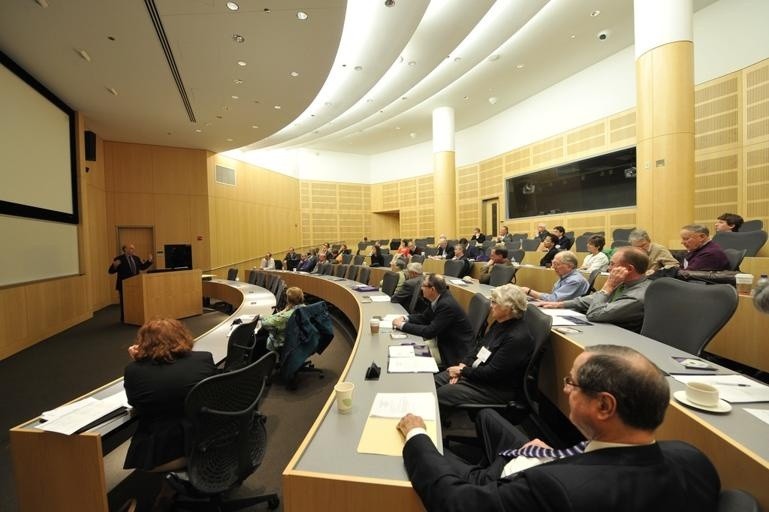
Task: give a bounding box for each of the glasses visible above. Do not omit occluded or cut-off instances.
[562,375,582,390]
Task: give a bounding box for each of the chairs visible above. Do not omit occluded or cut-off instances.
[272,237,436,296]
[370,219,768,512]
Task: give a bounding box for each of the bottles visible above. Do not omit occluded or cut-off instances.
[756,273,769,295]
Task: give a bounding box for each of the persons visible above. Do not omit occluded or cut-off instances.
[126,315,214,511]
[108,243,152,322]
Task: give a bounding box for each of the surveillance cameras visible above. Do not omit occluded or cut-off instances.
[597,31,608,41]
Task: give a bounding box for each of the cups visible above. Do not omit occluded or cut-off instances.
[734,273,754,297]
[334,381,355,415]
[370,319,380,336]
[293,268,297,273]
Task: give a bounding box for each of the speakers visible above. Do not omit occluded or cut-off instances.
[85,131,96,161]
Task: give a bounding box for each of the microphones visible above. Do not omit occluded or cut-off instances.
[143,259,152,272]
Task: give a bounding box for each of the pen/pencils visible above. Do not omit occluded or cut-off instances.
[715,382,751,387]
[400,341,415,346]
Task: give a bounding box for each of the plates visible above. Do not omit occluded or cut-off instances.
[671,390,732,415]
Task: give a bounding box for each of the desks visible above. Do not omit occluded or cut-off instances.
[9,268,443,512]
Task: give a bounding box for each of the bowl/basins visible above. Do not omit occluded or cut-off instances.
[689,381,722,404]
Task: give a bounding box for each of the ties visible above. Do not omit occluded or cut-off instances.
[498,439,591,459]
[128,256,137,275]
